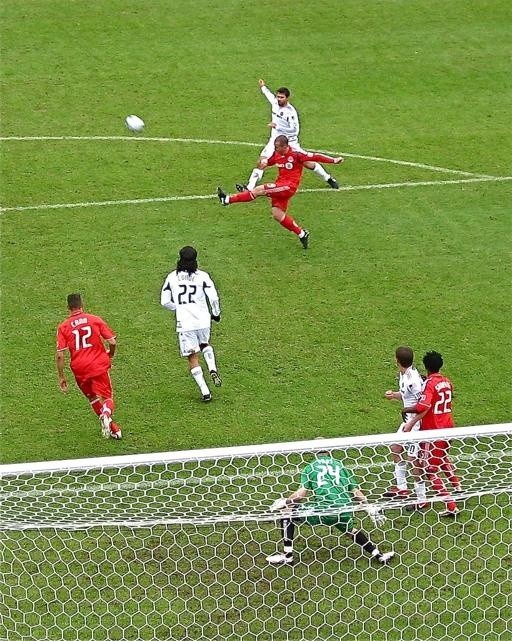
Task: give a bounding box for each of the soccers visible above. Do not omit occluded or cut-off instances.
[126,115,144,131]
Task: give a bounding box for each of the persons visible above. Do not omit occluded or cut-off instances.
[266,439,396,565]
[384,346,430,512]
[401,352,465,518]
[236,79,339,192]
[218,135,343,249]
[56,294,123,440]
[161,245,221,404]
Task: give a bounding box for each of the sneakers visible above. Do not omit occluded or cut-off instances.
[383,487,430,511]
[298,229,310,249]
[200,391,212,402]
[378,551,395,565]
[99,413,111,439]
[216,187,229,206]
[265,554,294,566]
[438,507,460,518]
[325,175,339,190]
[209,370,222,387]
[236,183,249,192]
[110,428,122,440]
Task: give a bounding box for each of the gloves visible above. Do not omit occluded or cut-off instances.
[269,497,293,512]
[365,505,388,528]
